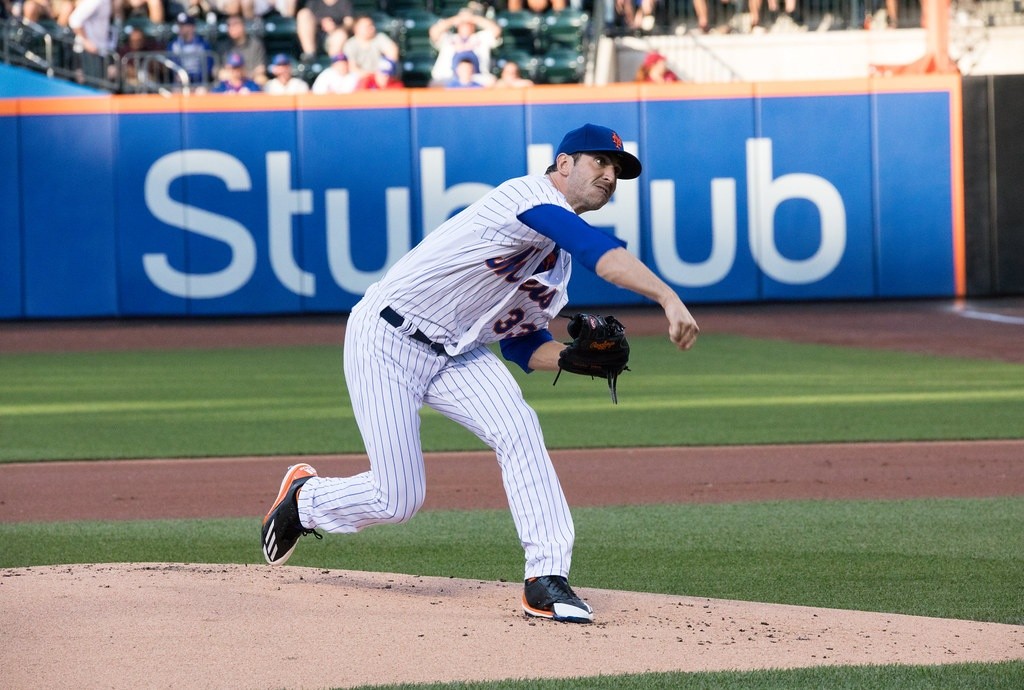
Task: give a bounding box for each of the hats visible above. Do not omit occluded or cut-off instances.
[553,123,641,179]
[272,54,287,66]
[225,53,243,67]
[331,54,348,64]
[377,58,395,74]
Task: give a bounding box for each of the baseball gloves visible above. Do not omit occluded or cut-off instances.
[557,311,631,381]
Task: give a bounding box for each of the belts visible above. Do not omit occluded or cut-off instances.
[379,306,446,354]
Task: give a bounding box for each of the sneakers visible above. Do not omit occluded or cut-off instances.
[522,575,593,623]
[262,463,319,565]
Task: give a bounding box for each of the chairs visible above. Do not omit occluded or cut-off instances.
[0,0,593,96]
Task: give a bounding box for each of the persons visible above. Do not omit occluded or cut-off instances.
[260,123,701,624]
[5,0,902,92]
